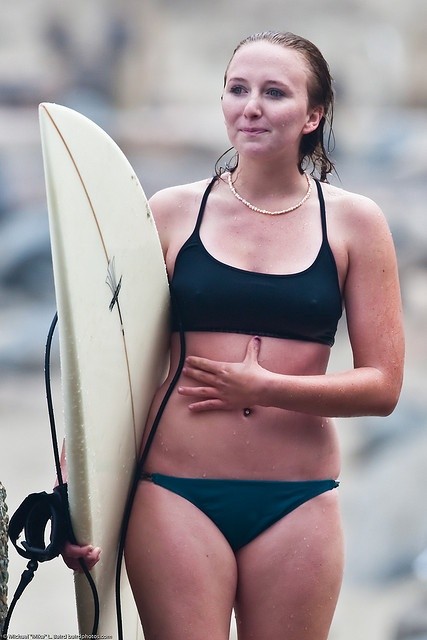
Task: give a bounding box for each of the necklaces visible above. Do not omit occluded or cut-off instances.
[227,170,314,216]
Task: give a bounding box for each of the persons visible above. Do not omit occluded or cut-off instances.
[54,31,405,640]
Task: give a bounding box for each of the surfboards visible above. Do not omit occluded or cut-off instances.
[37,102,171,640]
